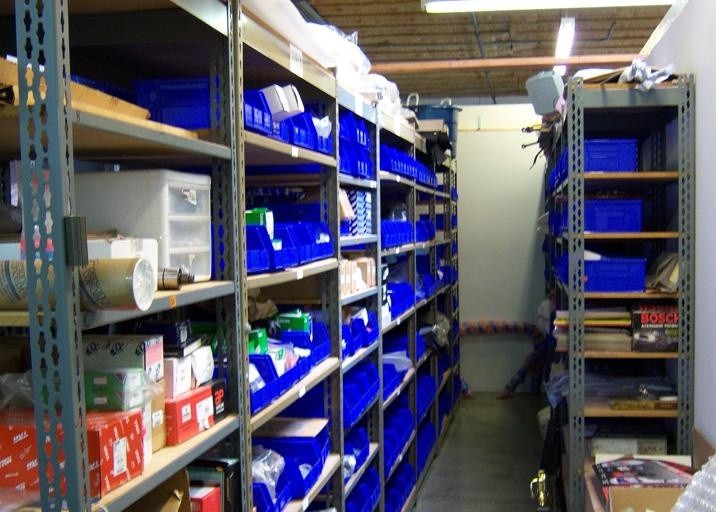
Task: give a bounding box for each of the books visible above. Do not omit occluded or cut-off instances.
[551,306,632,353]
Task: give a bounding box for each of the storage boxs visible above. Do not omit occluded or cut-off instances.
[283,109,314,148]
[267,440,322,500]
[585,426,715,512]
[130,73,269,135]
[211,378,227,415]
[544,138,647,296]
[348,468,377,512]
[1,1,247,512]
[362,367,378,401]
[361,308,378,345]
[75,170,213,285]
[263,427,332,457]
[246,224,268,273]
[348,109,368,141]
[309,221,334,259]
[248,335,311,389]
[349,428,368,466]
[353,142,376,176]
[2,230,160,278]
[316,108,333,152]
[158,357,190,396]
[343,388,362,426]
[141,472,190,512]
[139,317,202,357]
[387,146,459,510]
[309,320,334,363]
[167,378,214,444]
[219,357,275,413]
[287,222,315,265]
[344,322,362,358]
[263,222,298,269]
[32,368,143,411]
[269,116,284,141]
[190,345,214,383]
[80,333,163,382]
[1,406,145,502]
[339,135,351,175]
[254,456,293,512]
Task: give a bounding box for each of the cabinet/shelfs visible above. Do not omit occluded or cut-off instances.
[342,85,383,512]
[544,75,693,511]
[248,2,338,512]
[379,111,462,512]
